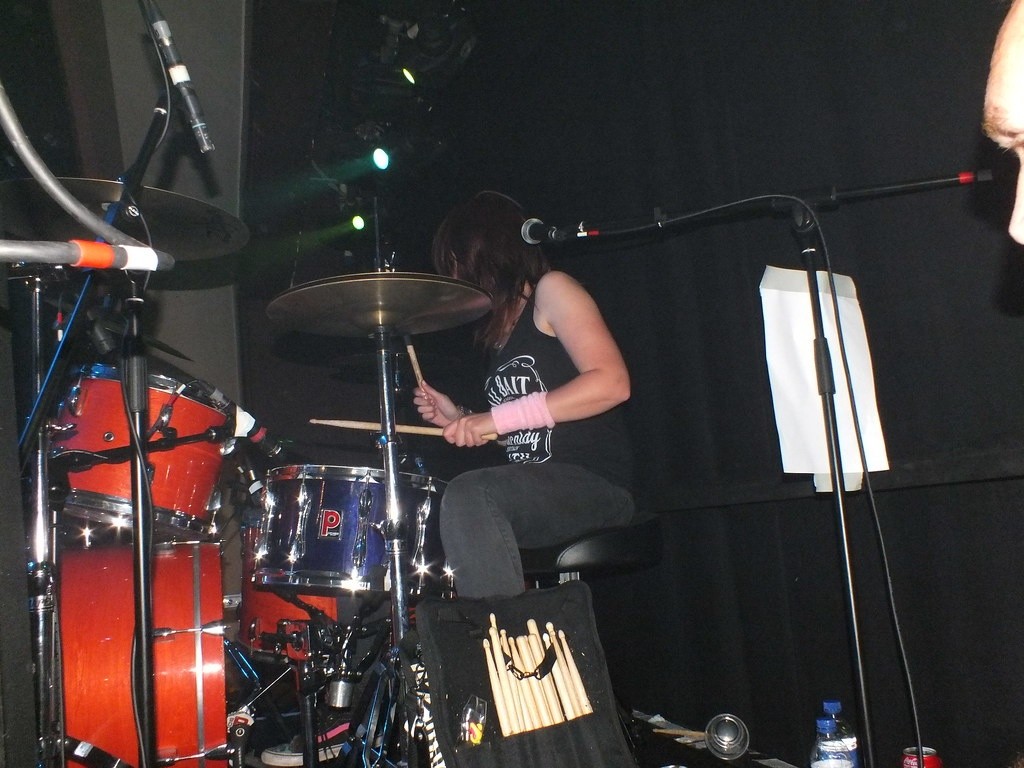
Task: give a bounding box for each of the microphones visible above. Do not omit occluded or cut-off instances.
[519,216,567,247]
[143,0,216,156]
[198,379,282,459]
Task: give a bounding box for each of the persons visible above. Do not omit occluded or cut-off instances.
[977,0,1024,246]
[412,191,637,598]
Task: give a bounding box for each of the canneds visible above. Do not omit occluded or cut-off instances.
[900,746,942,768]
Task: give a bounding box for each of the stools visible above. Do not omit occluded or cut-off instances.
[520,509,663,756]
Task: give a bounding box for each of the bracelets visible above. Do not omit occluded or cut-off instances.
[457,405,472,418]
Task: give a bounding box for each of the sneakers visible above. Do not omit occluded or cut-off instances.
[261,713,383,767]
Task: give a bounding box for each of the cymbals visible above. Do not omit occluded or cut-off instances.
[265,272,496,335]
[29,292,195,361]
[0,176,250,261]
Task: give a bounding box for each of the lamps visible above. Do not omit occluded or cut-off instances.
[306,0,484,256]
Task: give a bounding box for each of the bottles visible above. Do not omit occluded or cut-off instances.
[822,699,859,768]
[810,718,854,768]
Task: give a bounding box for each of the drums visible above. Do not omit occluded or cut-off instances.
[238,517,339,662]
[253,464,448,606]
[57,530,244,768]
[43,356,228,540]
